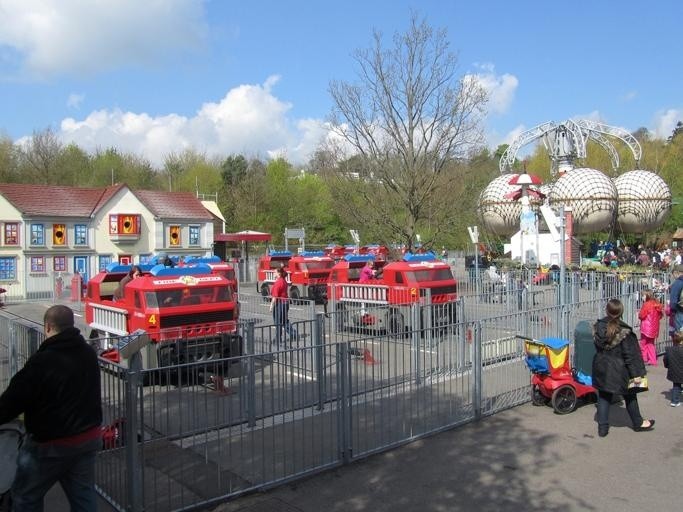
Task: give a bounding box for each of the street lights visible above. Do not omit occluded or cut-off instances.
[348,228,360,255]
[554,204,569,311]
[467,224,480,309]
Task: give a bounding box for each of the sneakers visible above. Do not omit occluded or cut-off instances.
[635,420,654,431]
[670,403,680,407]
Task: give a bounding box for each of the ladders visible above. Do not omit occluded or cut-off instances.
[339,283,389,304]
[263,270,292,283]
[88,303,129,337]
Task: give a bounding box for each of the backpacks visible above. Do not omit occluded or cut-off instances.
[678,288,683,307]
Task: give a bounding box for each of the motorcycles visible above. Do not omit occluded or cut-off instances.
[522,320,606,413]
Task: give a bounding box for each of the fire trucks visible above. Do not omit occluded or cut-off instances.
[81,253,244,386]
[253,246,460,335]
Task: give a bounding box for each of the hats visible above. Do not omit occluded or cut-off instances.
[673,264,683,271]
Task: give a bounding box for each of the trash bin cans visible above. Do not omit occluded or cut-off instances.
[574,320,597,377]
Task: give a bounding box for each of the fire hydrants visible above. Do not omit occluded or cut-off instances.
[54,277,63,298]
[65,272,86,302]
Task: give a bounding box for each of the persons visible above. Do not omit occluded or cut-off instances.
[112,265,142,300]
[638,290,662,365]
[0,305,103,512]
[669,265,682,333]
[663,333,682,407]
[359,259,376,279]
[605,243,682,271]
[269,268,300,342]
[592,298,655,437]
